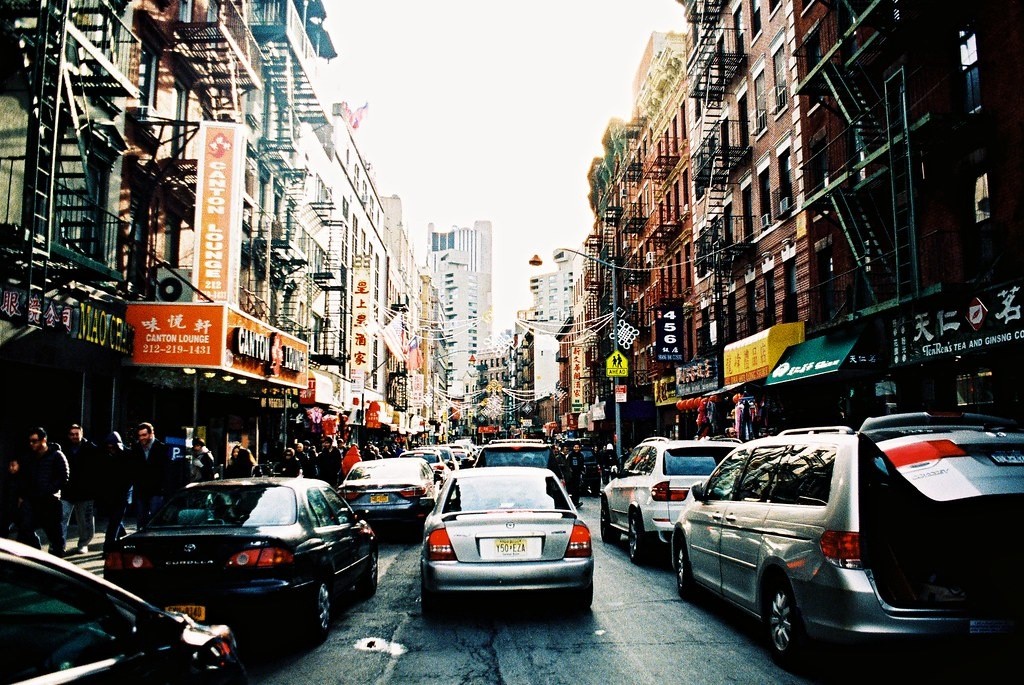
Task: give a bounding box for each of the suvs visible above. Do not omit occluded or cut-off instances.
[674,414,1024,670]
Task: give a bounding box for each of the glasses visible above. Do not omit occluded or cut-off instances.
[28,439,41,443]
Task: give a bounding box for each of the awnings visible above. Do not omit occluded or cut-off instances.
[765,327,879,386]
[702,382,744,397]
[578,413,588,429]
[723,321,805,387]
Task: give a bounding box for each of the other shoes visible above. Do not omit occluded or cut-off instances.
[77,545,88,553]
[576,502,583,508]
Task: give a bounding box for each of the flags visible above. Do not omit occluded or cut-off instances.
[406,337,422,370]
[383,313,406,362]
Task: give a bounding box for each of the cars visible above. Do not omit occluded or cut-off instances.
[105,478,378,634]
[413,448,459,471]
[474,439,567,493]
[443,439,482,465]
[399,451,452,490]
[338,458,435,530]
[423,466,593,612]
[600,438,747,561]
[0,538,246,684]
[565,447,600,493]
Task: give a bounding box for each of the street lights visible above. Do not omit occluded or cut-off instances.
[554,244,622,458]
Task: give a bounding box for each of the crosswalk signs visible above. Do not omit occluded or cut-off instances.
[607,352,628,377]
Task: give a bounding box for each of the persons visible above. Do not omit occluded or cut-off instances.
[0,422,739,557]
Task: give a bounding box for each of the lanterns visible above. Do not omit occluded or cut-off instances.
[676,393,744,412]
